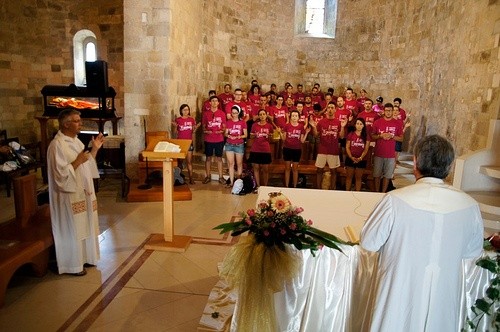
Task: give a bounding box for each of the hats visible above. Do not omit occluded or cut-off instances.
[376,96,383,103]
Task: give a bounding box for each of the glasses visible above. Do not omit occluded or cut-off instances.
[65,120,81,123]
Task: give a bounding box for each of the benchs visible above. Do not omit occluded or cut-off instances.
[0,127,54,305]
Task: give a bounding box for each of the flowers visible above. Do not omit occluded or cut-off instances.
[211,191,354,257]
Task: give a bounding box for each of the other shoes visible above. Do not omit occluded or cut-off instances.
[189,179,195,184]
[202,176,211,184]
[253,185,260,193]
[396,160,400,164]
[391,174,395,180]
[219,178,227,184]
[83,263,95,267]
[63,268,87,276]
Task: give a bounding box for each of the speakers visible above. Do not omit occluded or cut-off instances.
[85,62,108,89]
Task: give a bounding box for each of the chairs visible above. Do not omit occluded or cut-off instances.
[246,139,374,188]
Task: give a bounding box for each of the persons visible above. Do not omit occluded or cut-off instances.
[202,80,406,194]
[308,102,348,190]
[249,109,274,193]
[359,135,484,332]
[201,96,227,185]
[172,104,201,185]
[370,103,403,193]
[346,117,369,191]
[47,109,106,276]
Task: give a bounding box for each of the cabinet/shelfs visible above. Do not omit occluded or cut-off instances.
[42,86,118,137]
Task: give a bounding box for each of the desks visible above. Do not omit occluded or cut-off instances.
[231,186,500,332]
